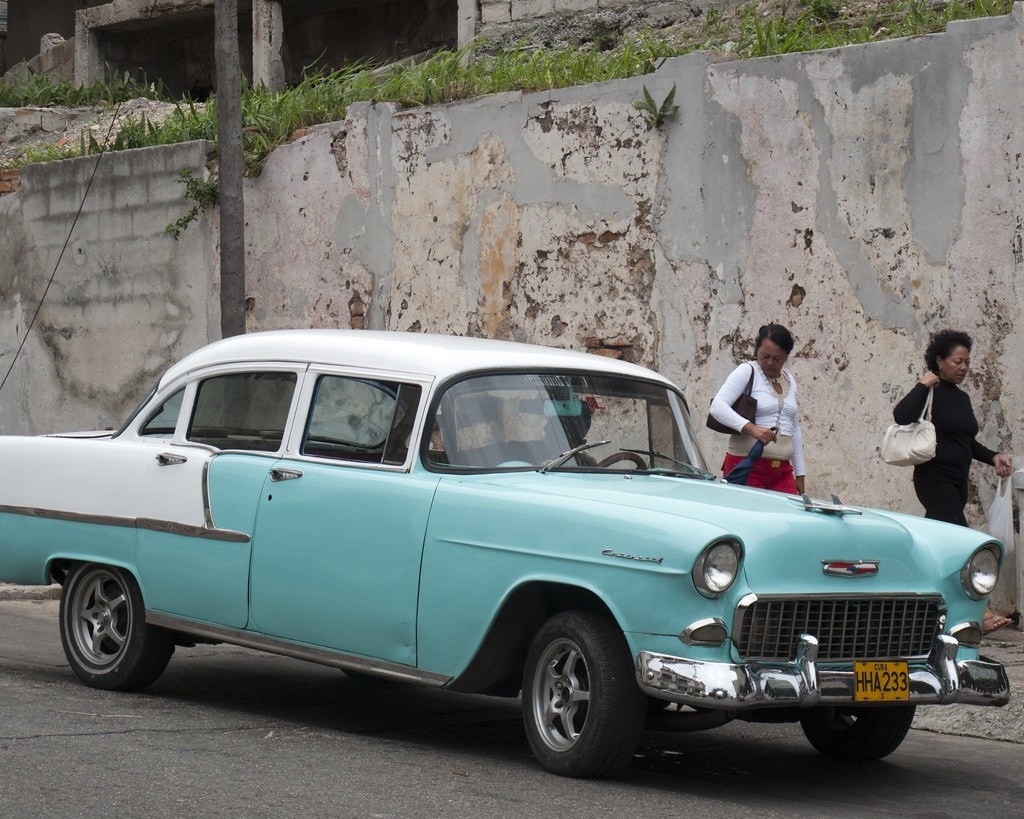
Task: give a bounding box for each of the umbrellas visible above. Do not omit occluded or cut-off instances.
[725,427,778,484]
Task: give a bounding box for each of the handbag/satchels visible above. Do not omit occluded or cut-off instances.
[880,382,935,466]
[985,471,1014,557]
[706,361,756,434]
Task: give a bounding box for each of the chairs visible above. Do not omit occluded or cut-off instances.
[372,410,447,463]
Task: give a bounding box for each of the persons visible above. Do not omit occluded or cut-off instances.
[894,330,1014,636]
[709,323,806,496]
[514,400,597,467]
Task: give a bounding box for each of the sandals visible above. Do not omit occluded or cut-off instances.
[981,617,1014,636]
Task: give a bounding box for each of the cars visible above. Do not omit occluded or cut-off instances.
[1,327,1013,786]
